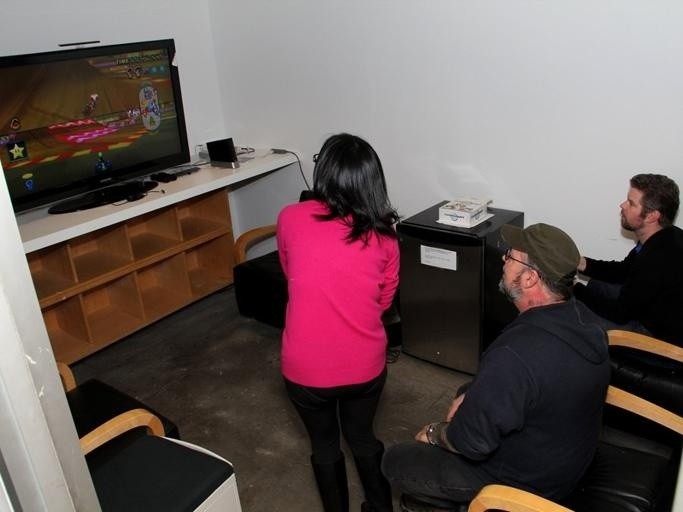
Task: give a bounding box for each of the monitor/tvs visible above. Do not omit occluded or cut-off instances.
[0,37,194,215]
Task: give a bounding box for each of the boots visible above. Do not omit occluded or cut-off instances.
[349,440,394,512]
[310,450,350,512]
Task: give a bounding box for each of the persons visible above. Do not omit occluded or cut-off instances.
[378,223,611,512]
[275,134,401,512]
[573,174,683,367]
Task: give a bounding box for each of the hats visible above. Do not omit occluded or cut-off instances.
[499,222,581,283]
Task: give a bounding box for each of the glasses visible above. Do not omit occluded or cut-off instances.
[313,154,320,162]
[505,246,541,280]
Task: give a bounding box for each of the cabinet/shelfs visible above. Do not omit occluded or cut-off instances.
[15,148,301,367]
[390,199,525,378]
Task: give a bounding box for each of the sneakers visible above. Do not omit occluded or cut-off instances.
[400,493,462,512]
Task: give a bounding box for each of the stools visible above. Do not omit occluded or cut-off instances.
[84,435,243,512]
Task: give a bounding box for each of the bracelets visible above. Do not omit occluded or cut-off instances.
[425,423,440,447]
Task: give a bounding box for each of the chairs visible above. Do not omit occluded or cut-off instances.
[233,190,321,331]
[56,361,181,481]
[468,385,683,512]
[606,329,683,377]
